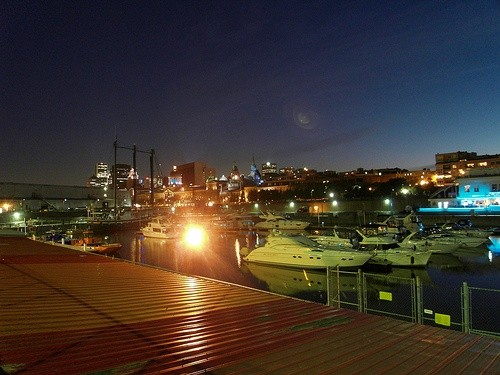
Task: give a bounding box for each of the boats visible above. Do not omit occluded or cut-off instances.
[243,229,377,268]
[350,229,432,266]
[434,221,499,238]
[430,233,488,248]
[139,213,183,239]
[372,212,425,233]
[252,209,311,229]
[30,226,123,256]
[397,231,463,254]
